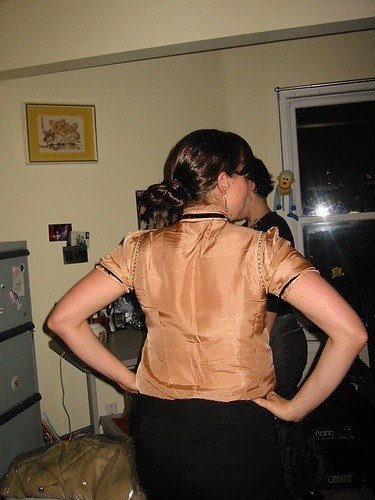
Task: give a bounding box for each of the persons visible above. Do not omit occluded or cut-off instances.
[46,129,369,500]
[235,157,327,500]
[52,225,86,263]
[117,320,122,327]
[139,206,181,231]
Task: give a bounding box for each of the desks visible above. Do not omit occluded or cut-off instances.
[47,323,146,441]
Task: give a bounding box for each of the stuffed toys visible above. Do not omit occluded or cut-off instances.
[272,170,299,221]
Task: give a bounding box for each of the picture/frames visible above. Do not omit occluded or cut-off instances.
[21,101,100,165]
